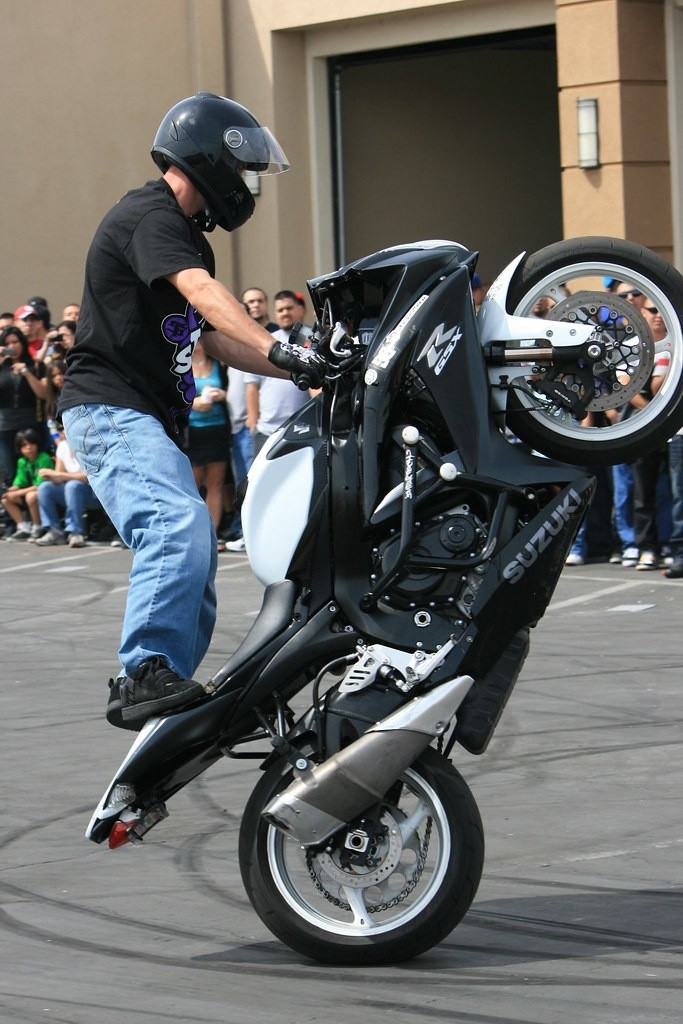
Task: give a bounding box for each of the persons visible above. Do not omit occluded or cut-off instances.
[1,277,683,579]
[55,90,330,730]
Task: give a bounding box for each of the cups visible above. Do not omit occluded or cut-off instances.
[200,386,217,404]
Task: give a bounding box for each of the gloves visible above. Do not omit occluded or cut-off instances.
[268,341,331,389]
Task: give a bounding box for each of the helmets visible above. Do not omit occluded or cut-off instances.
[150,91,270,233]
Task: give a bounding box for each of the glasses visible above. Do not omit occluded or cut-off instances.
[618,289,643,299]
[57,426,64,432]
[642,305,658,314]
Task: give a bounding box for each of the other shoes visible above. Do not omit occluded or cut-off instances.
[216,538,225,552]
[7,529,43,543]
[609,552,622,563]
[657,556,683,577]
[110,541,121,546]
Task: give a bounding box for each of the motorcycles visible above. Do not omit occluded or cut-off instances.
[82,235,683,950]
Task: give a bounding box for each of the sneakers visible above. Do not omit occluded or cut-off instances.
[225,536,246,552]
[68,532,86,547]
[636,551,657,570]
[621,548,640,566]
[36,527,67,546]
[105,656,205,732]
[565,554,584,564]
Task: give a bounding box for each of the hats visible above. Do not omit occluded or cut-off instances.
[604,276,615,288]
[14,305,38,321]
[29,309,50,322]
[29,297,47,307]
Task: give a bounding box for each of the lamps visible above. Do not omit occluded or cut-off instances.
[576,98,599,170]
